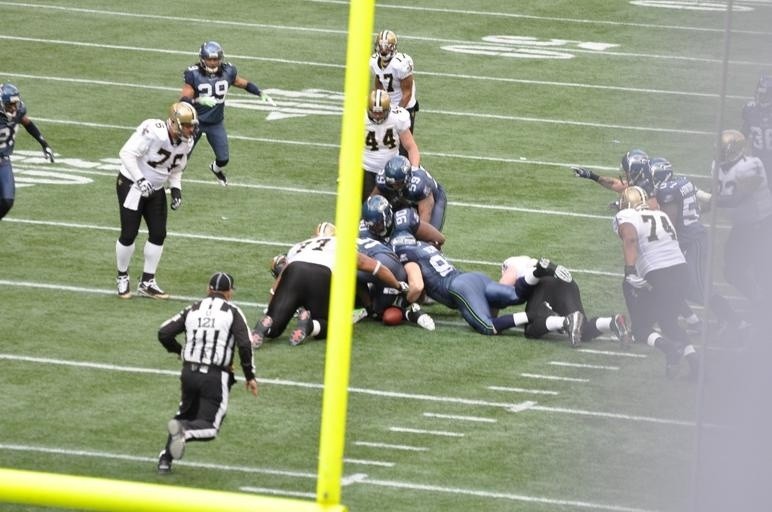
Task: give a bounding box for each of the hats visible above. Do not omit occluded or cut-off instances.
[209,272,236,291]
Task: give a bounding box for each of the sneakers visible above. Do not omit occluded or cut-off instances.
[158,419,186,473]
[537,302,585,347]
[208,162,227,186]
[610,314,632,345]
[410,302,435,332]
[117,275,130,299]
[138,279,169,299]
[537,258,572,284]
[252,309,313,350]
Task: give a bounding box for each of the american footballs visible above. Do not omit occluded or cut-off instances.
[381,307,402,325]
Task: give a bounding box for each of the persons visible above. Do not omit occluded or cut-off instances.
[250,154,447,349]
[113,100,201,301]
[0,83,54,217]
[178,42,278,191]
[573,124,770,387]
[368,30,420,159]
[487,253,635,348]
[361,89,421,202]
[382,230,574,337]
[156,271,260,473]
[742,71,770,191]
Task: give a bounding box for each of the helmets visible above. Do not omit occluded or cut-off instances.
[376,30,397,44]
[168,101,200,143]
[712,129,747,168]
[617,149,673,211]
[363,156,417,252]
[199,41,223,74]
[0,83,22,119]
[369,89,390,113]
[313,222,336,237]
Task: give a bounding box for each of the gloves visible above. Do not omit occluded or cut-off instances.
[258,94,275,105]
[171,188,181,209]
[43,144,54,163]
[138,178,155,197]
[625,273,648,289]
[398,281,409,293]
[196,96,217,108]
[571,166,599,182]
